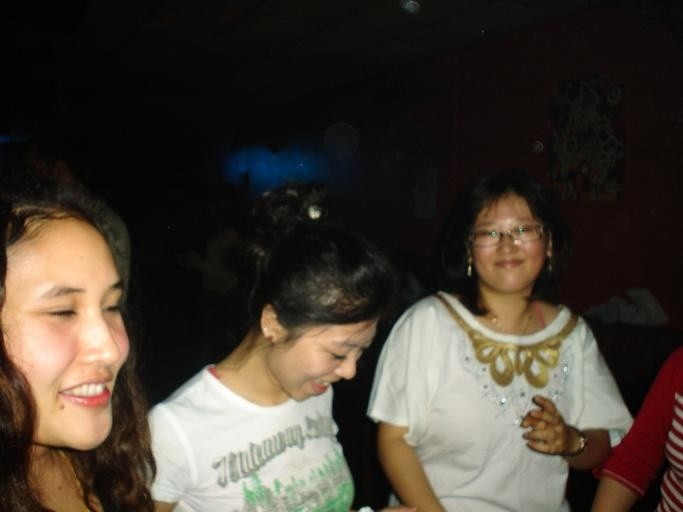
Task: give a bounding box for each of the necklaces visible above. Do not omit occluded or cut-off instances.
[486,306,534,337]
[59,447,92,511]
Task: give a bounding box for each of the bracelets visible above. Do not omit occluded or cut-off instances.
[564,422,588,458]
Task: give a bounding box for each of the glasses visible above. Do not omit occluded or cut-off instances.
[470,223,543,247]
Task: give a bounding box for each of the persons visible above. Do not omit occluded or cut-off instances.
[115,175,405,512]
[0,158,162,512]
[586,343,683,512]
[361,162,638,512]
